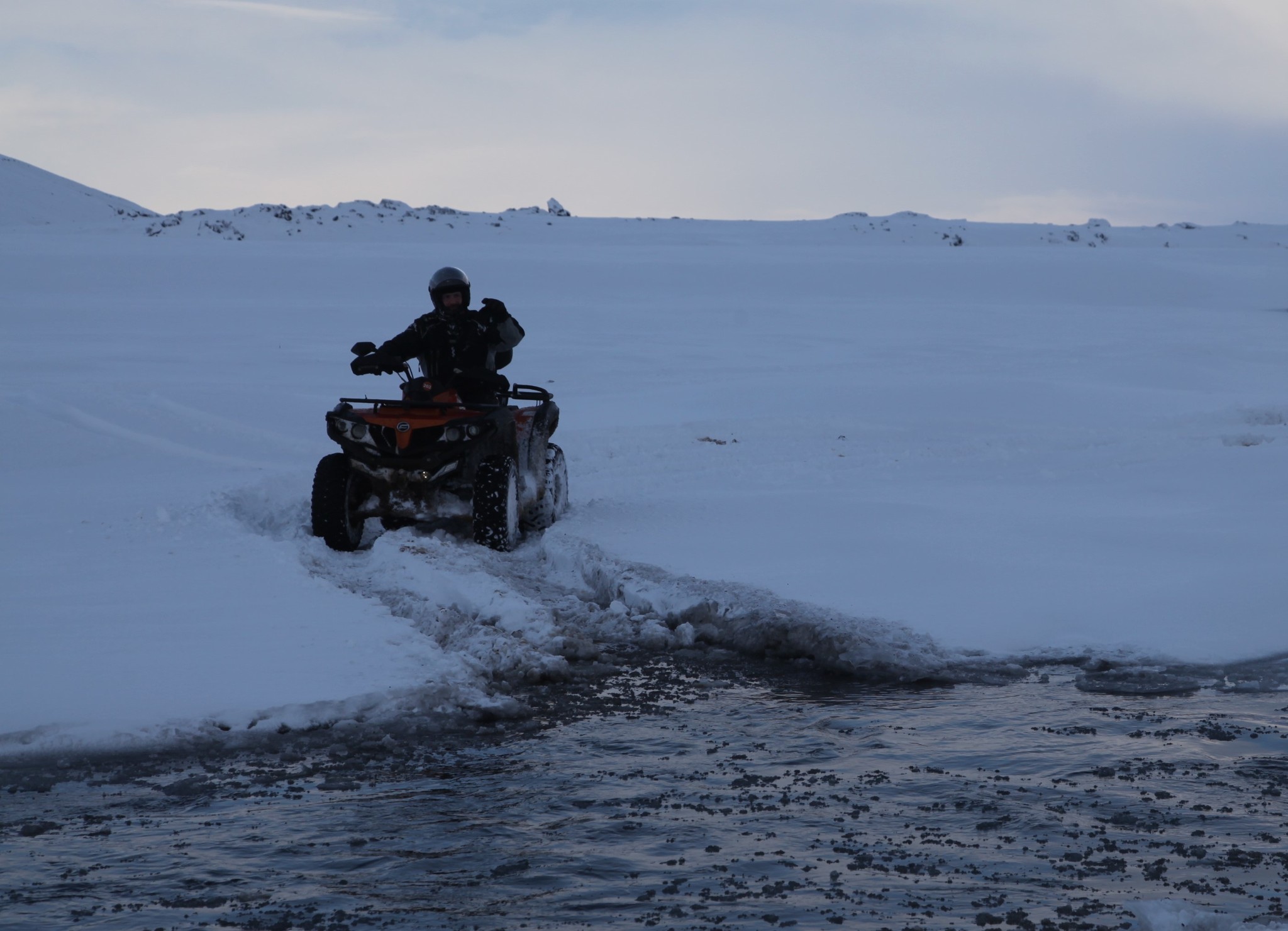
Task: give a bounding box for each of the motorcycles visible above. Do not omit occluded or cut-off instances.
[311,342,568,552]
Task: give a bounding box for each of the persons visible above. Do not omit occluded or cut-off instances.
[350,265,526,409]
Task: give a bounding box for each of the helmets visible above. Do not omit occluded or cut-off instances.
[428,267,471,309]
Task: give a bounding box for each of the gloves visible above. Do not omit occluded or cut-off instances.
[351,354,383,375]
[476,298,511,326]
